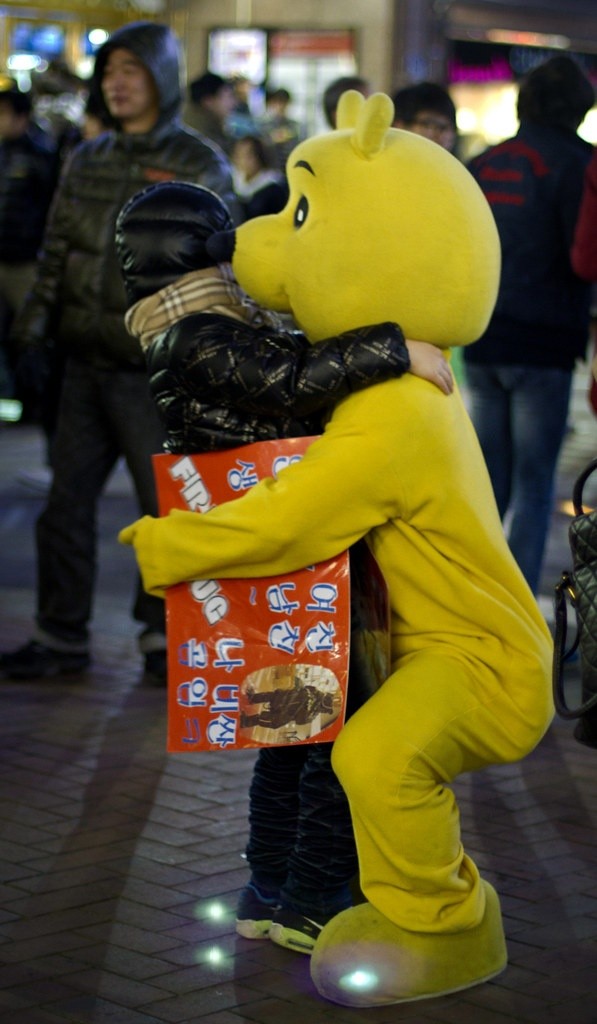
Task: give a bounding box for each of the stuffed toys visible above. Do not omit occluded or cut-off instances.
[116,90,556,1007]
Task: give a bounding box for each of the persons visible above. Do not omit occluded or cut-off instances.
[0,22,597,690]
[119,179,453,952]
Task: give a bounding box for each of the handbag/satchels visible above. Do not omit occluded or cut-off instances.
[552,459,597,747]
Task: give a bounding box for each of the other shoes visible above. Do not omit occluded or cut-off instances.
[269,893,357,954]
[236,885,277,940]
[138,628,167,686]
[0,633,95,684]
[535,590,578,626]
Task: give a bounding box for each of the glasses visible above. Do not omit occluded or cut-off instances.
[415,118,454,133]
